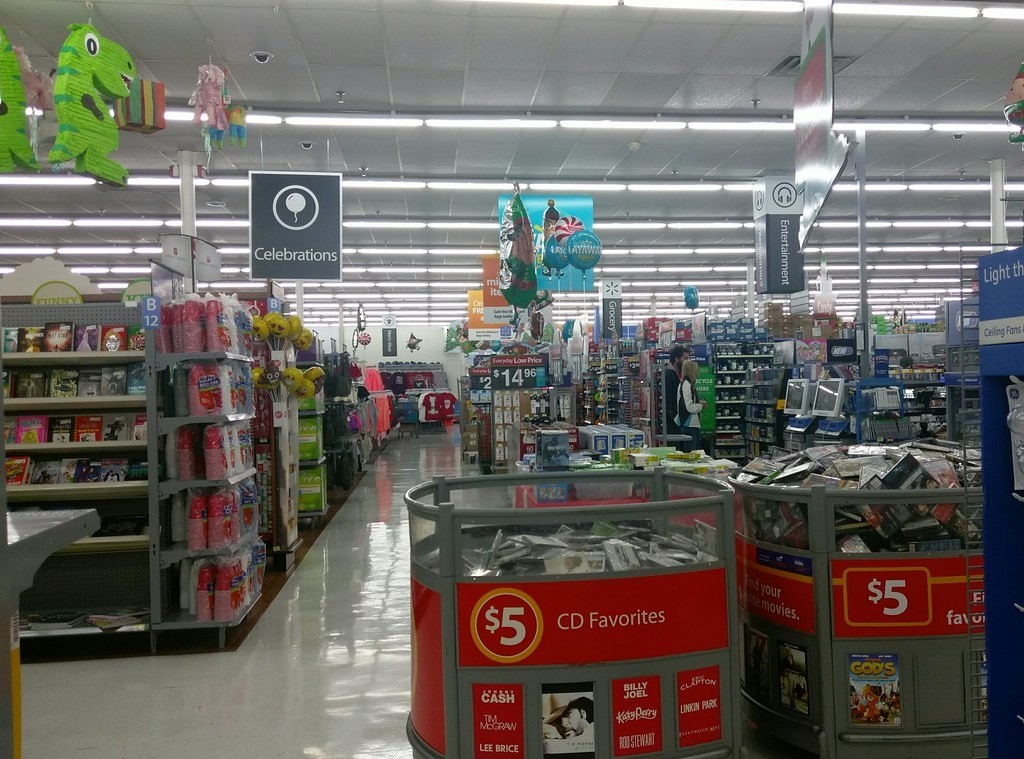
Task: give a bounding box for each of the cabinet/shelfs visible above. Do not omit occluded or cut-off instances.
[1,281,362,655]
[584,374,595,424]
[596,371,618,426]
[618,376,630,427]
[635,318,979,466]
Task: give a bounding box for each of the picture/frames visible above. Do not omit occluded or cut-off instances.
[381,327,397,357]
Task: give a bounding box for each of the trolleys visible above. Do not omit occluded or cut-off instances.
[398,401,418,439]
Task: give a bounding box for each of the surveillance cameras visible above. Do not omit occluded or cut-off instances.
[953,134,963,140]
[250,52,273,64]
[299,141,313,149]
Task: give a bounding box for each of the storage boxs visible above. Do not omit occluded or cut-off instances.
[297,466,326,509]
[464,423,478,465]
[297,415,324,461]
[297,367,320,414]
[504,418,646,499]
[630,301,886,351]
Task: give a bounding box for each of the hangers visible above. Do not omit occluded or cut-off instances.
[360,390,394,401]
[405,387,448,394]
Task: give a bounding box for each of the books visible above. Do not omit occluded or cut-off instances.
[19,607,151,630]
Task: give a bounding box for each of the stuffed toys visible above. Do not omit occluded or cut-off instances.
[189,65,230,129]
[201,89,249,174]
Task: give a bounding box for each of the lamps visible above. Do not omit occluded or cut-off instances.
[0,100,1024,322]
[303,322,643,325]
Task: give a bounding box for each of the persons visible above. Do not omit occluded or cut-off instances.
[664,347,690,448]
[564,556,589,573]
[543,693,594,740]
[677,360,707,453]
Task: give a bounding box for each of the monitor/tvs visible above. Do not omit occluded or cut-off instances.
[784,379,814,419]
[812,378,846,421]
